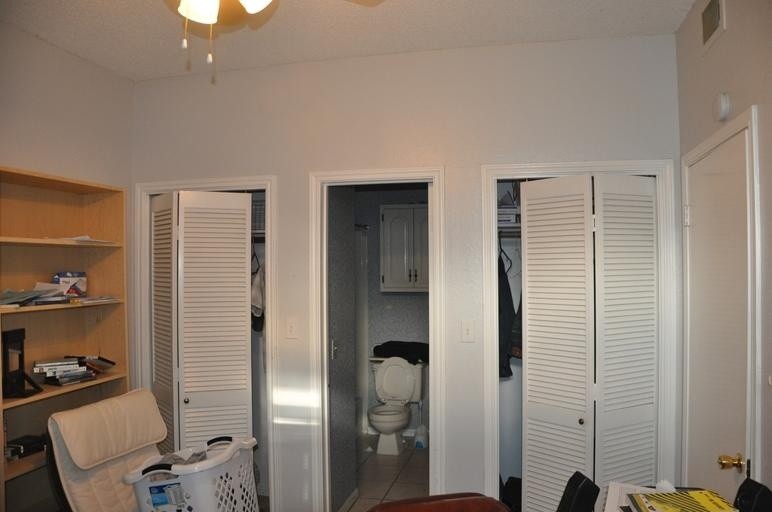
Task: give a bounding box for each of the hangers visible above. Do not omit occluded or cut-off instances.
[498,229,521,279]
[252,233,260,274]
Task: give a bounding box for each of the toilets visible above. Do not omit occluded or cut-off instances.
[366,356,424,457]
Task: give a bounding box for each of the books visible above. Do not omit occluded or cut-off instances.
[7,446,44,462]
[0,287,44,310]
[625,488,741,511]
[69,294,121,306]
[31,350,115,387]
[7,434,46,451]
[31,295,71,304]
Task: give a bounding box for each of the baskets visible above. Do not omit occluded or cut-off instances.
[125,436,260,512]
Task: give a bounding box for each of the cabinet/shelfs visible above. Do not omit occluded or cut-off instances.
[0,166,132,511]
[480,159,675,512]
[135,173,283,510]
[379,203,428,294]
